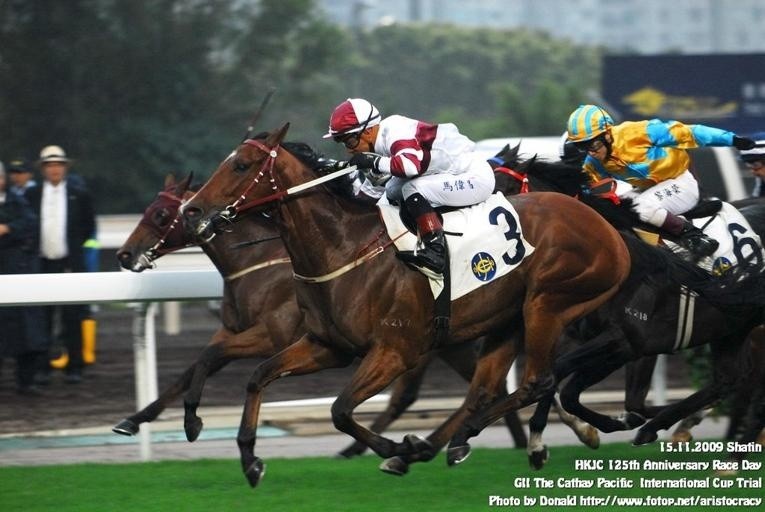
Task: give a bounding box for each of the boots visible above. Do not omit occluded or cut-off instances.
[396,192,446,281]
[653,212,720,260]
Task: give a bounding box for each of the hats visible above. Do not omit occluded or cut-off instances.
[323,97,382,140]
[736,131,765,162]
[38,145,73,164]
[565,104,614,146]
[6,156,33,173]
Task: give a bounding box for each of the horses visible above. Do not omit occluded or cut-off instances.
[108,120,765,489]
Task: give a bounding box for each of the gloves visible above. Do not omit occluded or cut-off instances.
[733,135,755,151]
[347,151,379,172]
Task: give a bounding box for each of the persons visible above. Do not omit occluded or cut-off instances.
[322,98,496,273]
[564,104,755,254]
[21,144,97,384]
[0,161,37,398]
[7,159,40,196]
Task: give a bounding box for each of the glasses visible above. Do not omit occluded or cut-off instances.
[333,133,361,150]
[744,160,765,170]
[575,140,604,153]
